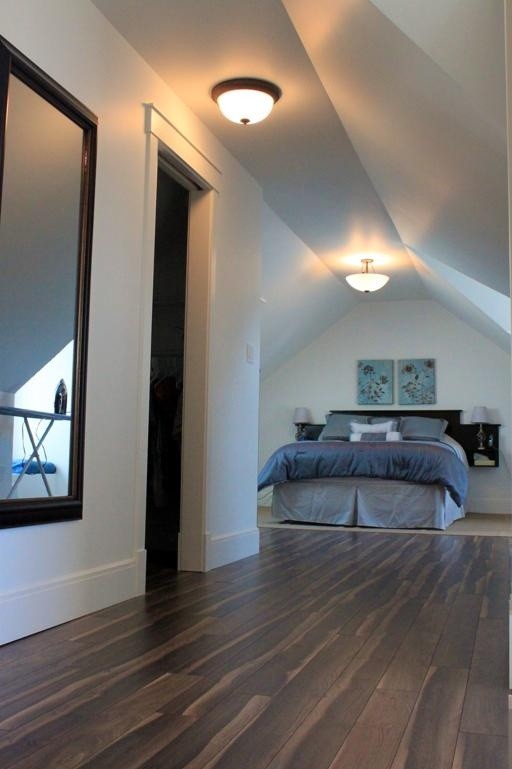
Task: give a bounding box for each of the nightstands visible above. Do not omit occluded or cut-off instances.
[469,423,501,467]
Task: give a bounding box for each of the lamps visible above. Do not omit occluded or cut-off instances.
[346,257,391,294]
[209,79,283,129]
[293,407,313,441]
[471,406,490,450]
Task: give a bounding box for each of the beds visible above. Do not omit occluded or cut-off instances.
[257,409,469,530]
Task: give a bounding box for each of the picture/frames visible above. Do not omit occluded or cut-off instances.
[357,357,437,406]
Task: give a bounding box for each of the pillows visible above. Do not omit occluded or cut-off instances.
[320,413,450,442]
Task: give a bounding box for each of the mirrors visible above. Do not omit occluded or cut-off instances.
[0,33,100,528]
